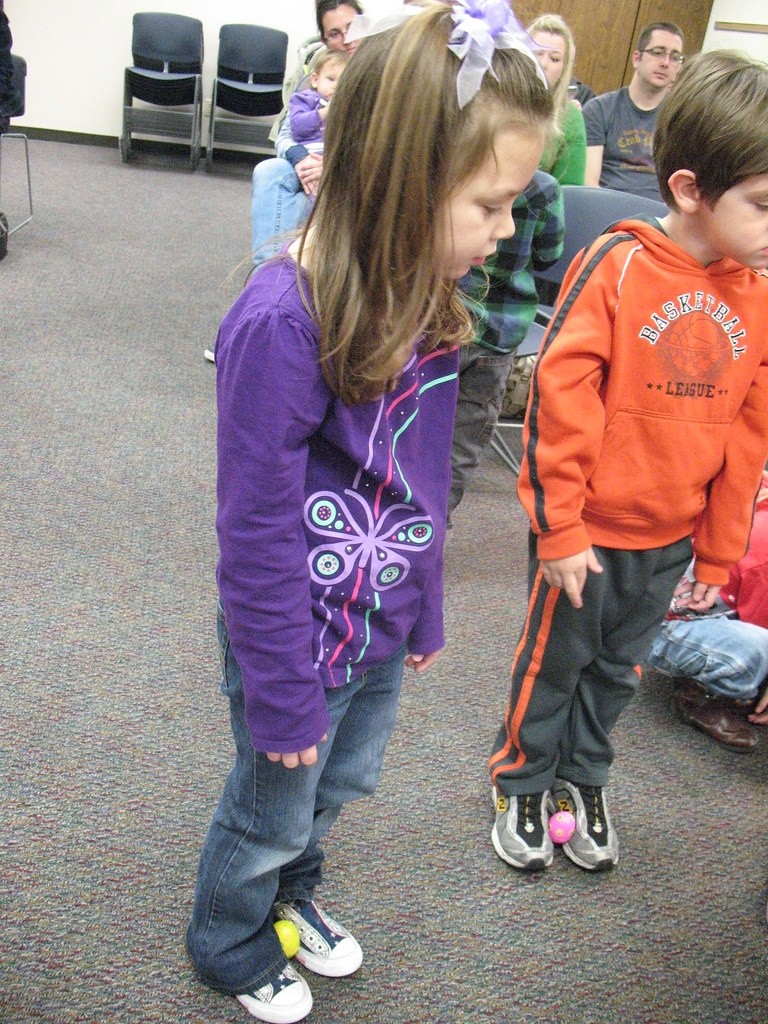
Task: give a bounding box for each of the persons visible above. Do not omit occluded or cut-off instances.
[180,7,549,1024]
[0,0,24,266]
[200,0,768,752]
[490,51,768,869]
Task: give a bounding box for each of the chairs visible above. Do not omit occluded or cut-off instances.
[489,185,670,474]
[121,10,290,174]
[0,55,34,234]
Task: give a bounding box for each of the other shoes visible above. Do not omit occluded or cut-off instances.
[204,344,216,362]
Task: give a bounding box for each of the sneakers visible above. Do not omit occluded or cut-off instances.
[547,781,619,869]
[491,785,553,868]
[274,896,363,977]
[237,962,313,1023]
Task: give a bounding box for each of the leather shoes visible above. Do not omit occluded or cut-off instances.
[671,681,757,752]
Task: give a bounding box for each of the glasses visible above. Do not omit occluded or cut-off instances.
[640,47,685,65]
[323,25,347,45]
